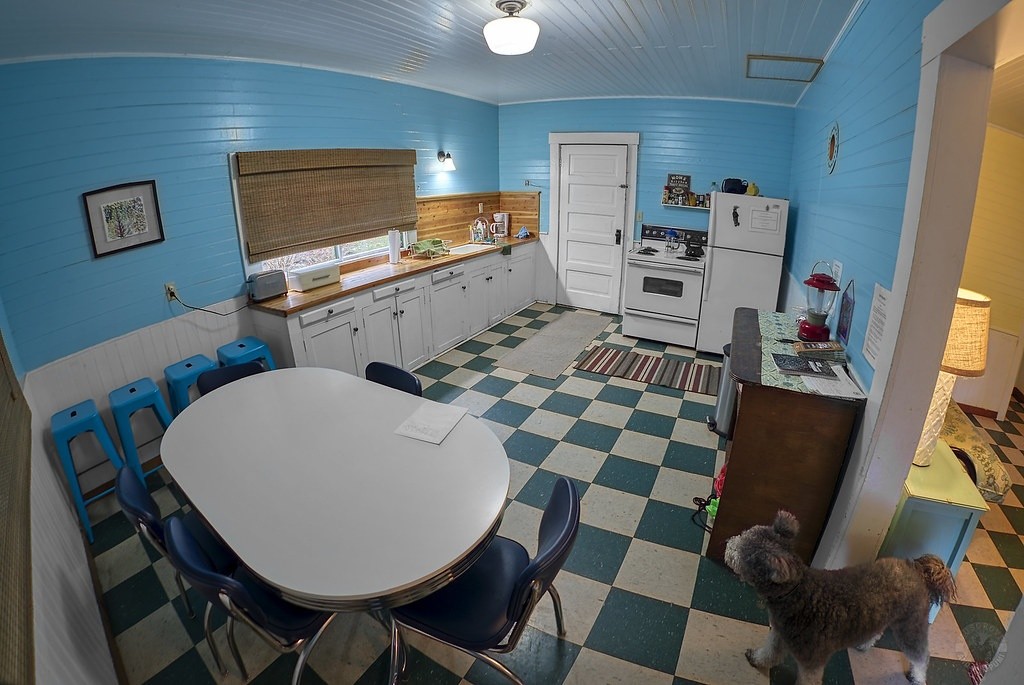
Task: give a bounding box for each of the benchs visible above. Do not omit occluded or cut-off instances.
[938,396,1012,504]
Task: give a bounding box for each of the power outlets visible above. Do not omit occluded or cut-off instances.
[525,180,529,186]
[164,281,178,302]
[478,203,484,214]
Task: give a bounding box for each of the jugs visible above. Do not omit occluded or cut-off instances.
[665,228,680,252]
[721,177,748,194]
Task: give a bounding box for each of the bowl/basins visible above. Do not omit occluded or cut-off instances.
[794,306,808,326]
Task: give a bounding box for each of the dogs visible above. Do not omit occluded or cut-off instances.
[725,511,958,685]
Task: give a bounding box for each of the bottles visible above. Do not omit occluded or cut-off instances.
[661,185,710,208]
[473,220,487,240]
[747,182,760,195]
[709,181,718,195]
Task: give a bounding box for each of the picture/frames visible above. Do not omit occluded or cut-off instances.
[82,179,165,258]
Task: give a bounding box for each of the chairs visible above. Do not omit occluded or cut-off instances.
[163,517,408,685]
[390,477,580,685]
[196,360,265,398]
[365,361,422,397]
[115,464,240,621]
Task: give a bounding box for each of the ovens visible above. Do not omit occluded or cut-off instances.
[622,258,704,348]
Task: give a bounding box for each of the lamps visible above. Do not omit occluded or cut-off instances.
[483,0,540,55]
[798,260,841,342]
[912,287,992,467]
[438,151,457,171]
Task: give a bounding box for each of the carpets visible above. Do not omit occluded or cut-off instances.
[572,344,722,397]
[491,310,613,380]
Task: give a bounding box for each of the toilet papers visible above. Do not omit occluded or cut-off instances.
[387,229,401,264]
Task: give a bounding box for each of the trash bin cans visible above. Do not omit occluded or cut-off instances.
[706,343,738,440]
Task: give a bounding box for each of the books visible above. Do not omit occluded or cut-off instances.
[794,342,847,366]
[771,353,839,380]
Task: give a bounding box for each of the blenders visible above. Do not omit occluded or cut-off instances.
[798,259,841,342]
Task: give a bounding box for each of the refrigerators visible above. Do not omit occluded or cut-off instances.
[695,190,790,353]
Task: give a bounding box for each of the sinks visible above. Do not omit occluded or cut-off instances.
[447,243,495,254]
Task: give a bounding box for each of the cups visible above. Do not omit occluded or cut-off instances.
[474,233,479,239]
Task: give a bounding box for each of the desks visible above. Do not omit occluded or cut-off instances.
[874,436,991,624]
[705,307,868,565]
[159,366,510,685]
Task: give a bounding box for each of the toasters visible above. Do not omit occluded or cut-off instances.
[245,268,288,302]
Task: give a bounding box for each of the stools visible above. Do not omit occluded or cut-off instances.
[216,335,277,381]
[163,353,225,417]
[108,377,174,488]
[50,399,124,543]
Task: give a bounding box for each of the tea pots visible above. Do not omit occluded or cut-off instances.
[683,236,705,256]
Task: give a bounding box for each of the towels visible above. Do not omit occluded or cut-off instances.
[496,241,512,255]
[409,238,447,256]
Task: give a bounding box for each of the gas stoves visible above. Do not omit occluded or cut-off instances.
[628,223,708,268]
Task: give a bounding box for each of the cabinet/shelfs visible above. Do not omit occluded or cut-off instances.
[426,260,470,357]
[506,243,536,317]
[251,292,368,379]
[468,251,507,337]
[358,271,431,372]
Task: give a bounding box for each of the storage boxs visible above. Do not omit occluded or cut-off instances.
[289,262,341,292]
